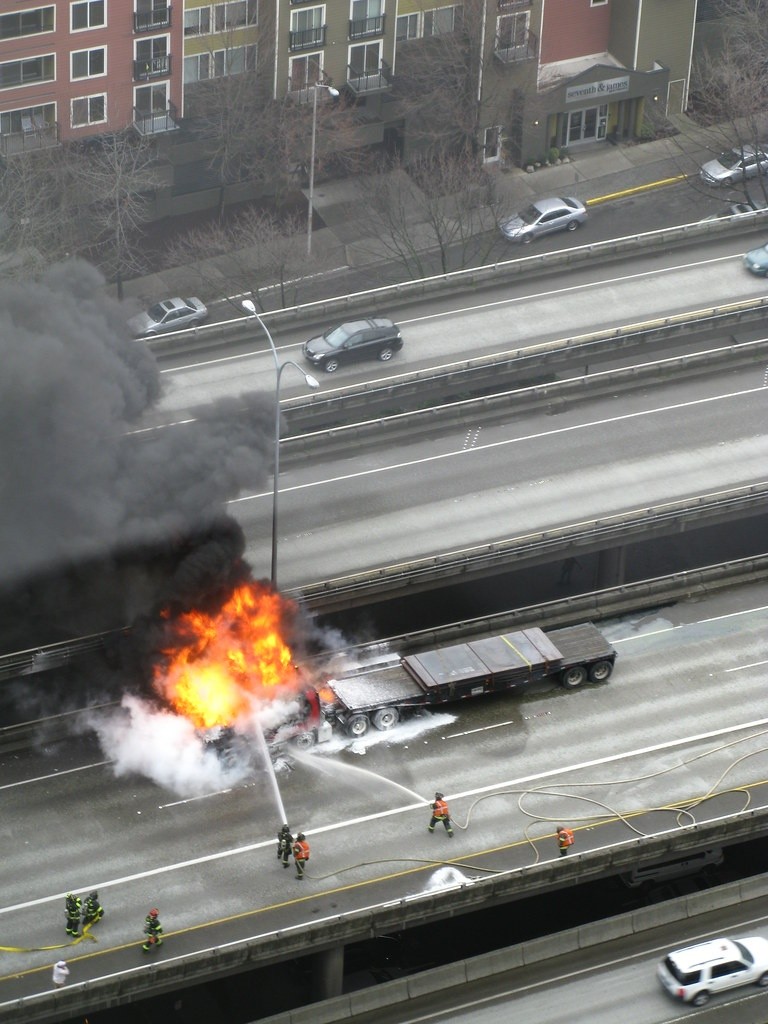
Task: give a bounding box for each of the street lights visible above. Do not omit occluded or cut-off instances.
[242,299,320,593]
[307,83,340,255]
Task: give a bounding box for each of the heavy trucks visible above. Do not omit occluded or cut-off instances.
[204,622,618,770]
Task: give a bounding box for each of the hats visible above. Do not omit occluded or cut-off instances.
[57,961,66,967]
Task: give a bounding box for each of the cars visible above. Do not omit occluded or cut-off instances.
[498,196,588,245]
[743,243,768,279]
[302,317,403,373]
[126,297,208,338]
[698,202,767,227]
[698,144,768,189]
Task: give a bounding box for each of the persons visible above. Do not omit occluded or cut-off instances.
[51,961,70,989]
[64,890,105,938]
[276,824,309,881]
[141,908,164,955]
[427,793,454,839]
[555,825,576,859]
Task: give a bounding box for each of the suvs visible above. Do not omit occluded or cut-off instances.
[659,936,767,1006]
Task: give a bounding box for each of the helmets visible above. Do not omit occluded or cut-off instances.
[436,793,443,798]
[90,890,98,899]
[282,826,290,833]
[557,827,563,833]
[297,834,306,842]
[150,908,158,917]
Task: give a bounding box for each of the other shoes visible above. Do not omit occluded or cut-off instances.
[284,864,290,868]
[295,876,302,880]
[73,935,81,938]
[448,832,454,838]
[278,855,281,859]
[155,943,164,949]
[143,949,151,954]
[428,827,434,833]
[65,931,72,935]
